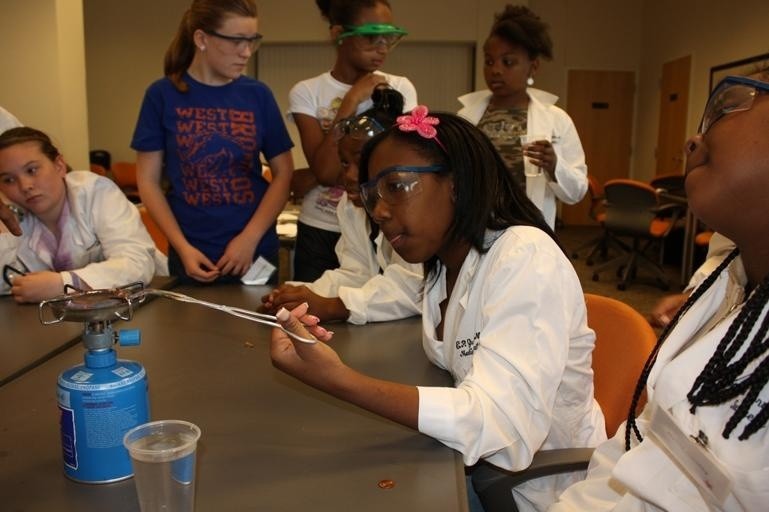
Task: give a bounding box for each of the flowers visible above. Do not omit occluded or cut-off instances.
[396,105,441,140]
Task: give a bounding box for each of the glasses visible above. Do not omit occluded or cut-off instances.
[206,31,261,52]
[696,77,768,132]
[2,264,27,289]
[338,24,407,50]
[358,167,457,213]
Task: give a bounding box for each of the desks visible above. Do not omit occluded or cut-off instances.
[1,276,473,511]
[1,275,191,388]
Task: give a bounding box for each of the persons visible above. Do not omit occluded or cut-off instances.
[130,1,297,288]
[542,51,769,512]
[283,0,423,284]
[454,6,589,235]
[0,127,157,305]
[0,199,23,237]
[258,110,609,510]
[645,234,736,329]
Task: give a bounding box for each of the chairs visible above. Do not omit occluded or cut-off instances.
[571,175,714,293]
[89,148,301,287]
[473,291,659,512]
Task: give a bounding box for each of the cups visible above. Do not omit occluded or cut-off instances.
[518,133,548,177]
[122,419,201,512]
